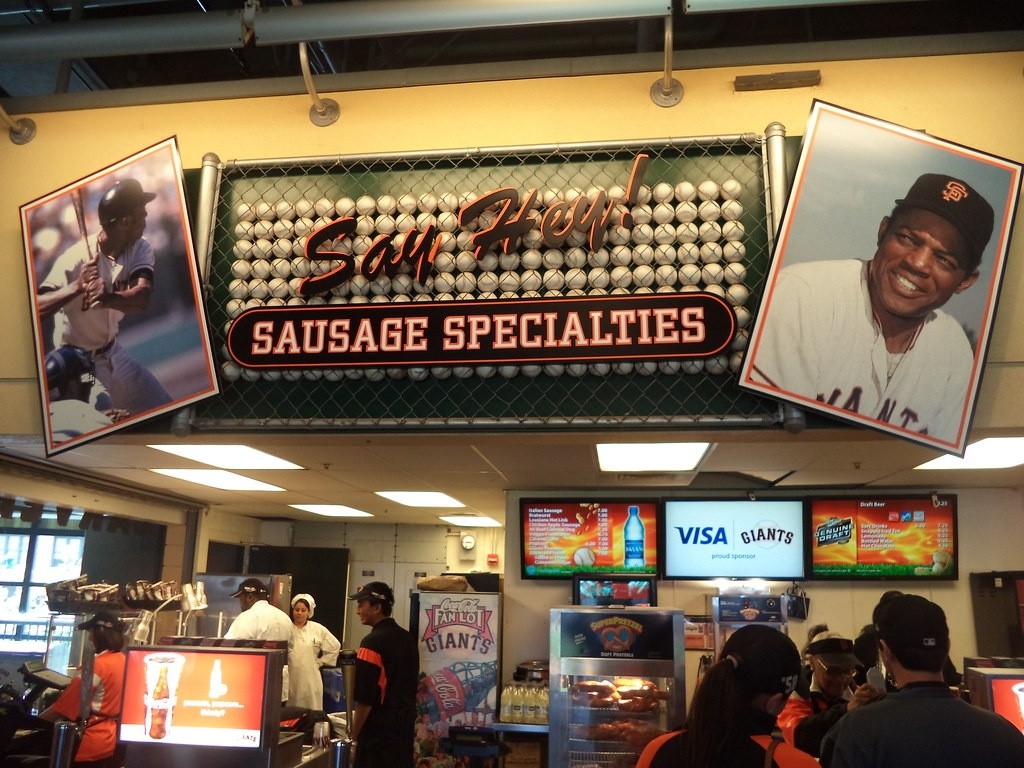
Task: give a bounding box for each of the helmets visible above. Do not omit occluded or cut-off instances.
[45,344,97,402]
[98,179,157,224]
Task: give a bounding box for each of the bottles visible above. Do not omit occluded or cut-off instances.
[499,684,549,725]
[624,507,645,571]
[150,667,169,739]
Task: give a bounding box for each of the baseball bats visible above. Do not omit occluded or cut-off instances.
[71,186,104,311]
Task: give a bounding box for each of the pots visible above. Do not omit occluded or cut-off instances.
[513,659,549,685]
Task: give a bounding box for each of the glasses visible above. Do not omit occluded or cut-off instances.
[814,659,856,677]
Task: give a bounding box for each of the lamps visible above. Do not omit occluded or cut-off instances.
[120,518,130,535]
[151,582,199,617]
[93,515,103,531]
[183,581,208,636]
[107,517,120,533]
[79,512,93,530]
[124,609,152,643]
[0,499,16,520]
[21,503,44,524]
[56,507,73,527]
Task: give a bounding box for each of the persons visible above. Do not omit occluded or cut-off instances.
[750,173,994,444]
[285,593,341,711]
[38,611,126,768]
[635,623,822,768]
[777,591,960,758]
[347,582,420,768]
[224,578,293,709]
[36,175,175,446]
[830,594,1024,768]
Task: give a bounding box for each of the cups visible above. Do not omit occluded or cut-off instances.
[144,653,184,740]
[313,722,330,749]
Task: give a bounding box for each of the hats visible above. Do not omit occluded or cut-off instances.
[809,631,853,651]
[896,173,994,262]
[291,594,316,618]
[230,578,268,599]
[78,611,124,631]
[348,582,395,606]
[863,595,948,646]
